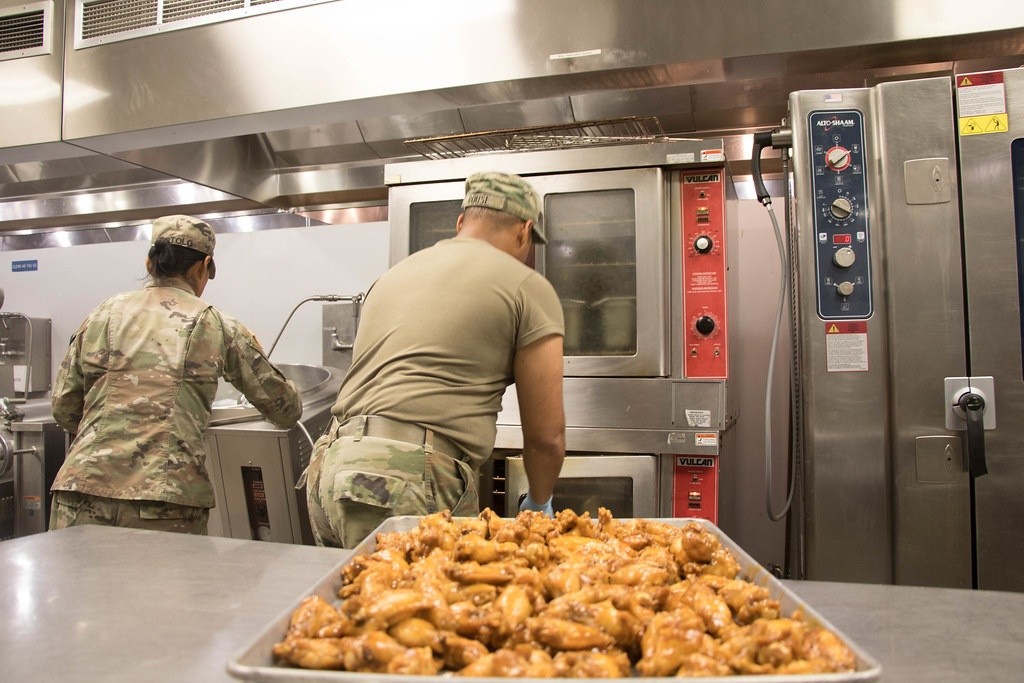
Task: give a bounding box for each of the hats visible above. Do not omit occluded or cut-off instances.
[151,214,216,280]
[461,171,549,244]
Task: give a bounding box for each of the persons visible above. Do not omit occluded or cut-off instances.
[294,171,566,551]
[48,214,303,536]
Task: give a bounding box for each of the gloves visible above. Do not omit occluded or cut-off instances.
[519,488,555,519]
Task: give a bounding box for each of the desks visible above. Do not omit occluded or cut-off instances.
[0,523,1024,683]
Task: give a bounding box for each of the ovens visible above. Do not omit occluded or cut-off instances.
[380,115,744,555]
